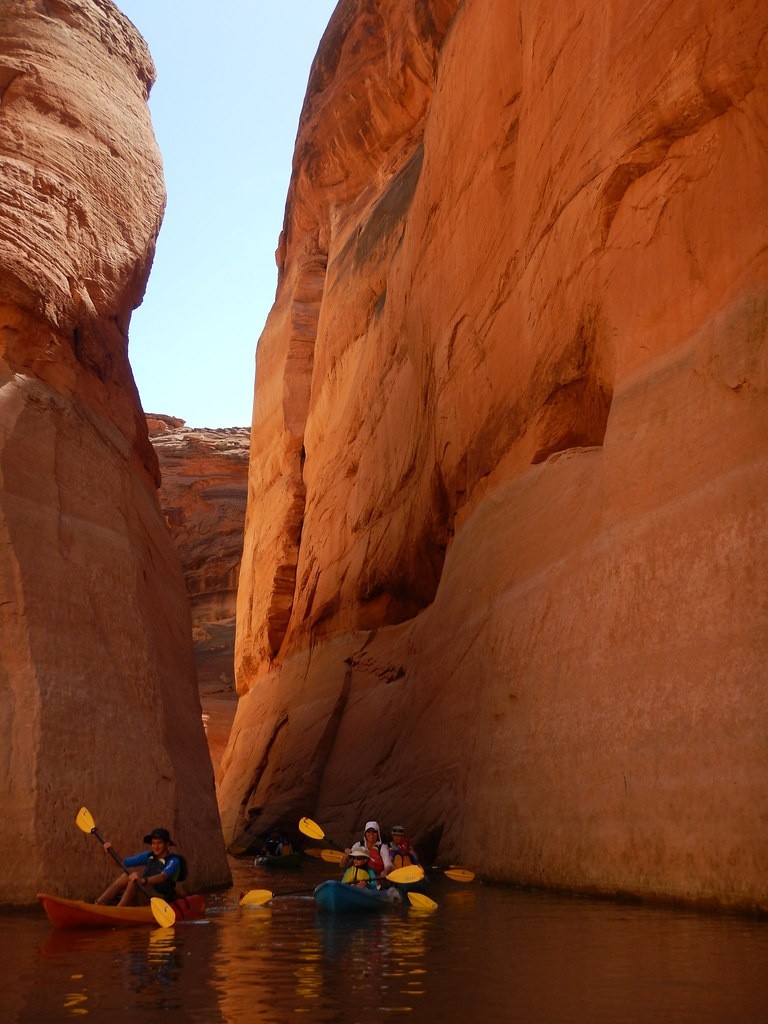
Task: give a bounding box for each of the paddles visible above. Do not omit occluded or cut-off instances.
[298,816,438,911]
[74,806,177,929]
[303,847,471,870]
[320,848,476,883]
[240,864,426,906]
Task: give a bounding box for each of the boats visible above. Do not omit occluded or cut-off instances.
[35,891,209,930]
[261,847,311,872]
[316,880,402,916]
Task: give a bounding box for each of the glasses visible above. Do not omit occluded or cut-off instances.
[366,828,377,833]
[352,856,366,860]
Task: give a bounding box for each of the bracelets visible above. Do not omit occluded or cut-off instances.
[141,876,148,886]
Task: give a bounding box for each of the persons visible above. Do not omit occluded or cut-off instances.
[345,822,394,878]
[262,823,294,858]
[341,846,377,892]
[384,825,419,870]
[94,828,180,907]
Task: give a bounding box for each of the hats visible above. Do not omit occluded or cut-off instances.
[391,826,405,835]
[347,847,375,862]
[144,828,176,847]
